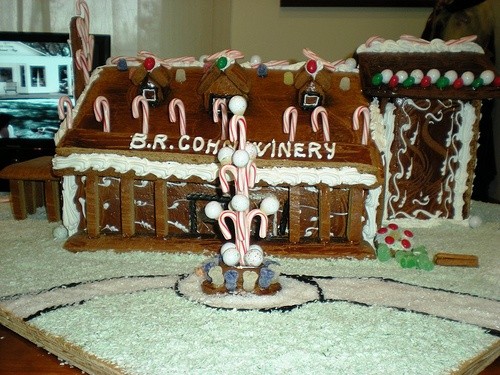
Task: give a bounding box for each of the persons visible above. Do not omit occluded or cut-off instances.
[420,0,500,204]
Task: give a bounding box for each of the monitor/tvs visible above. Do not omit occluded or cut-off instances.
[0,31,111,161]
